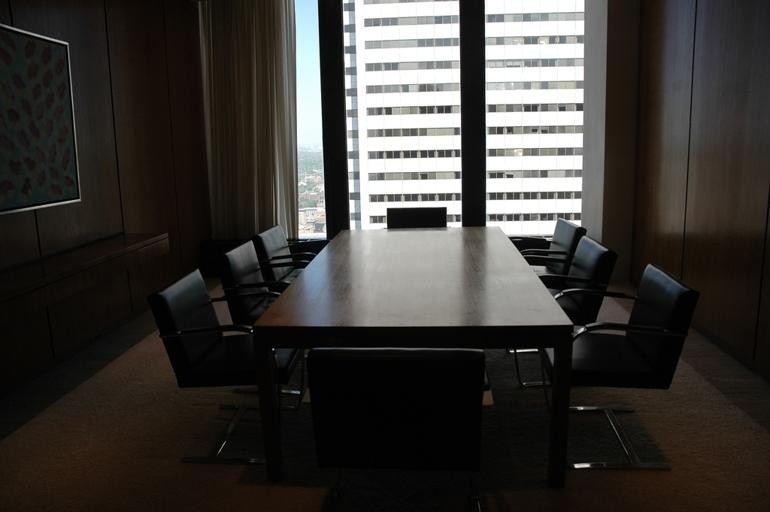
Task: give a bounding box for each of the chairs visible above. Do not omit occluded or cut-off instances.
[507,217,617,389]
[151,225,333,472]
[307,347,486,512]
[545,262,701,470]
[386,207,447,229]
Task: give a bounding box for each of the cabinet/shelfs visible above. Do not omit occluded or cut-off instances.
[630,2,768,381]
[2,230,176,397]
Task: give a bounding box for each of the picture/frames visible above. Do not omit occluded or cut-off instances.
[0,22,86,215]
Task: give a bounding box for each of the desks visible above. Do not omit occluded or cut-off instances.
[254,230,574,461]
